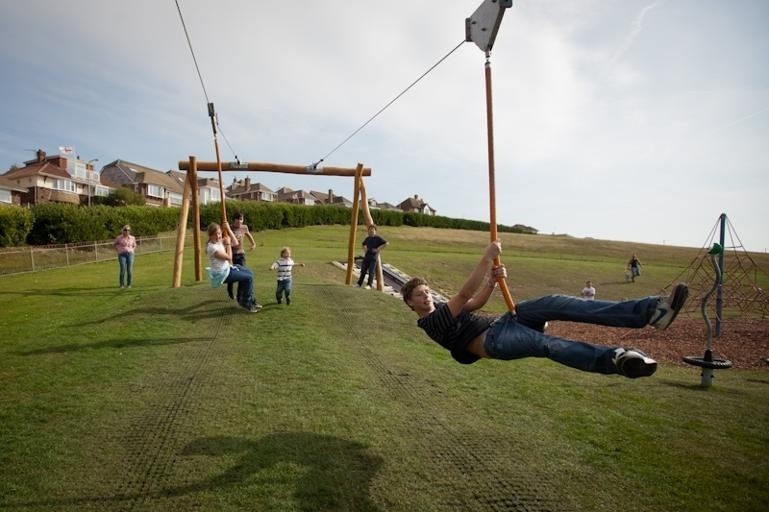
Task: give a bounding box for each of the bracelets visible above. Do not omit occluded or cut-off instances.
[487,278,497,288]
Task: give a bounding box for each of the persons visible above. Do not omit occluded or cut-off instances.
[628,252,644,282]
[268,246,305,306]
[205,211,262,313]
[353,225,389,289]
[580,281,596,301]
[401,241,689,379]
[112,225,137,289]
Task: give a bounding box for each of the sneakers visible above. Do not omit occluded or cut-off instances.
[609,343,659,379]
[353,282,373,290]
[645,277,690,331]
[241,304,263,314]
